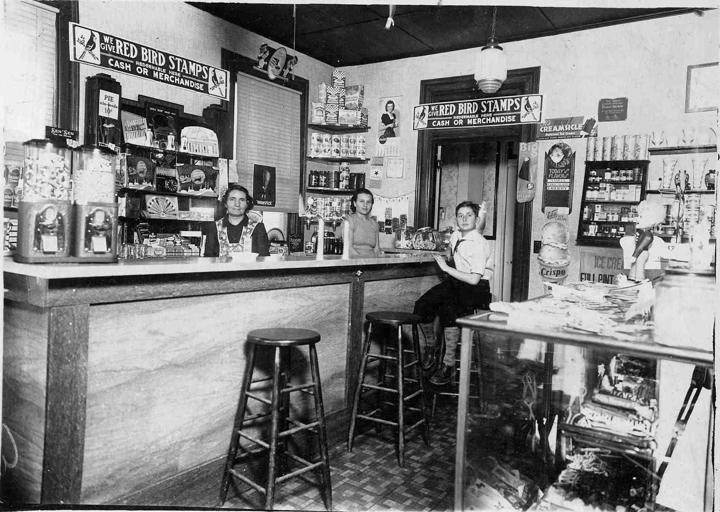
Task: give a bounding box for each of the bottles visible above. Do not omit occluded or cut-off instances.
[310,162,366,190]
[312,132,369,160]
[583,166,638,238]
[585,134,647,162]
[305,194,356,220]
[329,238,343,254]
[120,243,148,261]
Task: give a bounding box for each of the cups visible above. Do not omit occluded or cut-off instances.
[658,157,677,192]
[690,157,706,191]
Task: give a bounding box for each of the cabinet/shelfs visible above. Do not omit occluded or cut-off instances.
[303,110,373,198]
[574,158,651,250]
[83,73,235,262]
[644,141,717,279]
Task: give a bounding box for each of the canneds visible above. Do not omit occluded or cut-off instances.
[306,196,354,218]
[308,171,365,188]
[305,241,313,255]
[311,132,366,158]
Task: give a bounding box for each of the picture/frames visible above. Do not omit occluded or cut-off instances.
[683,61,720,114]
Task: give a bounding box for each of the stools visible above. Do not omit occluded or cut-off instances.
[427,305,492,434]
[216,325,335,512]
[341,310,432,468]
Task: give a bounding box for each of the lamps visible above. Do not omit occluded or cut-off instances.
[469,6,511,96]
[253,44,299,81]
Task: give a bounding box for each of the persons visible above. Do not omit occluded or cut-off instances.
[629,198,668,285]
[412,200,492,385]
[340,188,382,255]
[203,184,270,256]
[380,100,399,138]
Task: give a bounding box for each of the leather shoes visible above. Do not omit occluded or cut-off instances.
[429,362,458,386]
[422,345,440,370]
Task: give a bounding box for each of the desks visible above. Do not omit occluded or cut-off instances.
[450,280,715,509]
